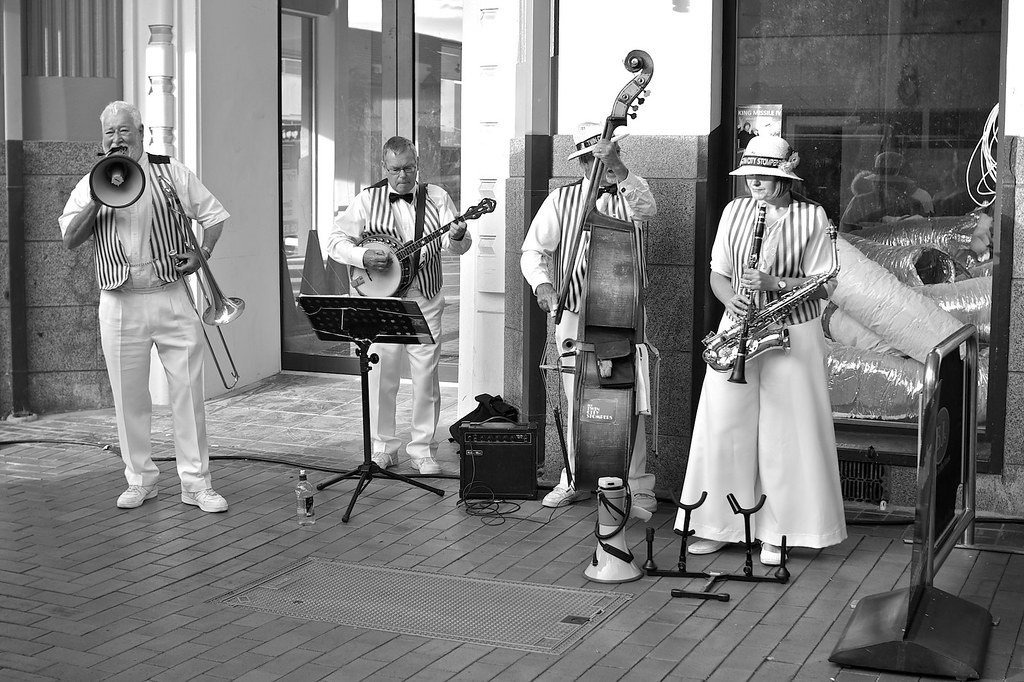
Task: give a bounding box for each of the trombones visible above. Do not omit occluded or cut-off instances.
[150,163,246,389]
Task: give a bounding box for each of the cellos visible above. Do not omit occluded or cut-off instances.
[539,49,643,500]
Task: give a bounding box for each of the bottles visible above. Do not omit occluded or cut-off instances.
[294,469,315,525]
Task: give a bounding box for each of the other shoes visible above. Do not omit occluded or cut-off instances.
[758,539,788,564]
[688,539,727,554]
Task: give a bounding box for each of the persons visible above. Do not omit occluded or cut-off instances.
[59,100,231,512]
[520,122,661,513]
[327,136,472,474]
[738,121,985,233]
[674,136,847,565]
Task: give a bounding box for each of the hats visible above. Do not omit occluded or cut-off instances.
[863,152,914,183]
[729,136,804,181]
[568,121,631,161]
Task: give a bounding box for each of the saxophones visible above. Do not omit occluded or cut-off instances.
[700,218,842,374]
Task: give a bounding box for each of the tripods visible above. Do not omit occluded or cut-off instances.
[294,295,445,523]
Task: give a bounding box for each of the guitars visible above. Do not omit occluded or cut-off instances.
[346,198,497,297]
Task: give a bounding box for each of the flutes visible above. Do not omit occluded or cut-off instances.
[727,205,767,384]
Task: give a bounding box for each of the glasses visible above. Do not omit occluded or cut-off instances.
[384,159,417,175]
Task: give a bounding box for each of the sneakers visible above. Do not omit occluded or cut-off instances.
[182,488,227,512]
[630,490,658,511]
[116,485,159,508]
[410,454,442,474]
[372,450,398,469]
[542,484,592,507]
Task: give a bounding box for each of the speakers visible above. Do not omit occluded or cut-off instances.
[459,421,540,500]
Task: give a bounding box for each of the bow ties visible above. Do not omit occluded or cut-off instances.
[389,193,413,204]
[597,184,618,199]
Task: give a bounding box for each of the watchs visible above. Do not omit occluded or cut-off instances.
[201,246,213,257]
[777,276,788,293]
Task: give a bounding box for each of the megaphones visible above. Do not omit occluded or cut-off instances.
[580,476,645,584]
[88,146,146,209]
